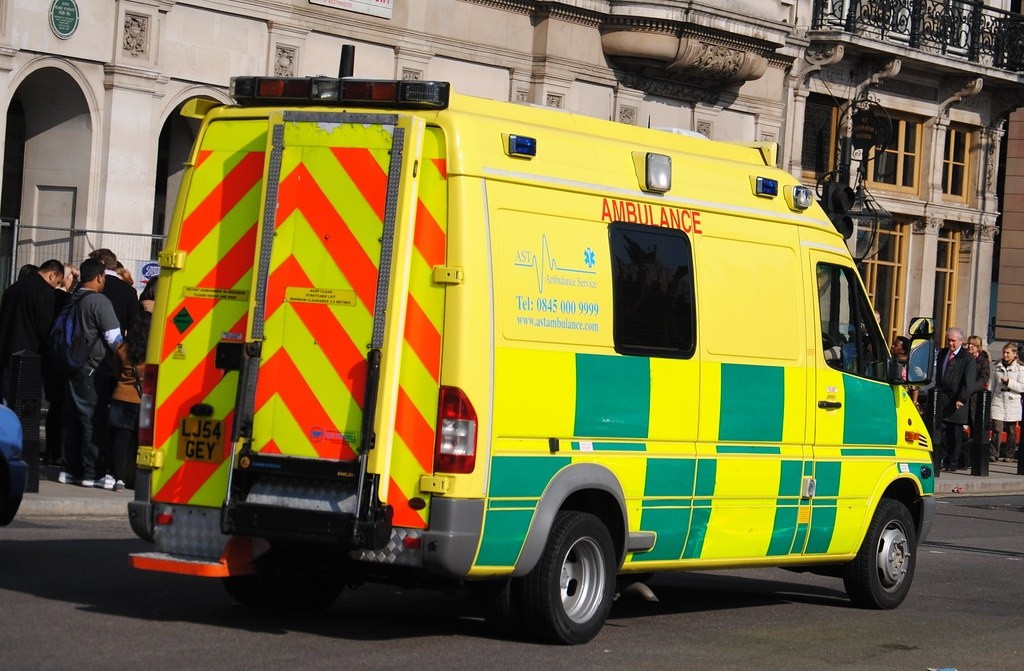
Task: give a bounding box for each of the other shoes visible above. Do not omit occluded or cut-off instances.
[944,466,970,471]
[1003,458,1013,463]
[989,456,996,462]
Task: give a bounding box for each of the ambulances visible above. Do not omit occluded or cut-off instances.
[128,74,934,646]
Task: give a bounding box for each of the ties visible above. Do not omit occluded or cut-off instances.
[946,352,955,368]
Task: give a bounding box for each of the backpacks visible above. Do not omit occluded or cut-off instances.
[47,290,102,372]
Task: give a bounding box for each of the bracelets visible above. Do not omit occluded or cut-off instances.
[921,371,925,379]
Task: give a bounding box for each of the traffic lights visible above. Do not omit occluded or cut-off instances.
[821,180,855,240]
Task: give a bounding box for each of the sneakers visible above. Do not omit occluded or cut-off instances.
[78,479,95,487]
[95,474,116,489]
[114,480,126,492]
[59,472,78,483]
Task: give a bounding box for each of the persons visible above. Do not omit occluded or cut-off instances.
[824,346,841,361]
[849,309,881,379]
[914,319,929,335]
[889,327,1024,472]
[0,249,161,491]
[914,366,927,378]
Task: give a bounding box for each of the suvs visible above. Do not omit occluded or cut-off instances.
[0,397,28,525]
